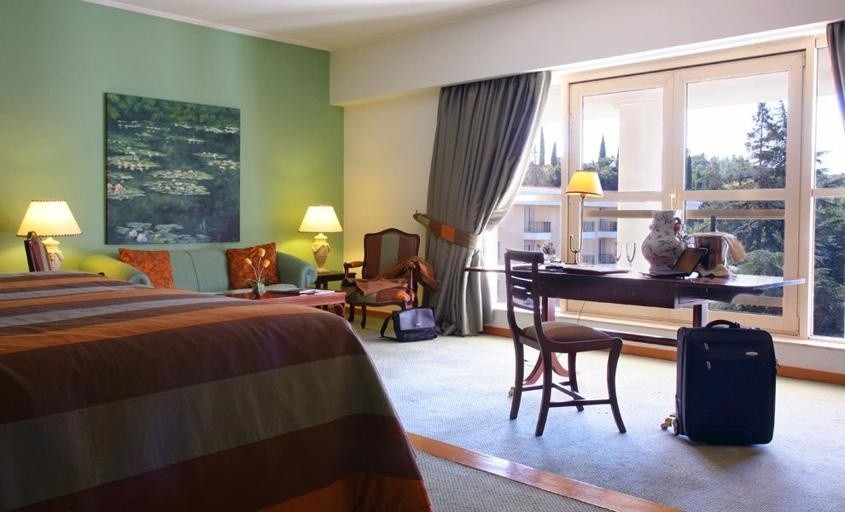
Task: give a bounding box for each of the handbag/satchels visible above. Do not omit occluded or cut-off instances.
[380,305,437,343]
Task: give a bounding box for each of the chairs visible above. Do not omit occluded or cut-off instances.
[504,250,626,437]
[341,228,420,329]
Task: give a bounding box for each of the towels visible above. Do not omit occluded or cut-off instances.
[686,232,746,263]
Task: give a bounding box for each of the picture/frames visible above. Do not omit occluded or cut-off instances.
[102,92,241,246]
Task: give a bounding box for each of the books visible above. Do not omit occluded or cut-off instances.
[286,288,335,296]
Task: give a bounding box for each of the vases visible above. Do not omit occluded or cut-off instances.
[252,282,265,296]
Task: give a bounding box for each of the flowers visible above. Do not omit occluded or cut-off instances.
[244,248,271,284]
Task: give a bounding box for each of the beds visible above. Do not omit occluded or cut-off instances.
[0,270,433,512]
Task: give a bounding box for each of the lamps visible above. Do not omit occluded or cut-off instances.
[565,169,603,252]
[299,206,343,273]
[16,200,82,271]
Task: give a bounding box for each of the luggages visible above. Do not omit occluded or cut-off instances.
[670,320,778,444]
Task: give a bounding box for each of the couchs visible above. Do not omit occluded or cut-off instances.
[82,242,318,299]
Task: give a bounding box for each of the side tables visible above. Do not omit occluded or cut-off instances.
[315,271,356,322]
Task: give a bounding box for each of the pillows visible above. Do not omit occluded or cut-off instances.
[226,242,278,289]
[118,248,175,288]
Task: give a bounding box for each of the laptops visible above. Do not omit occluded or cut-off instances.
[638,247,708,277]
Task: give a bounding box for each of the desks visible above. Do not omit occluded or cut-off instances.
[461,260,805,428]
